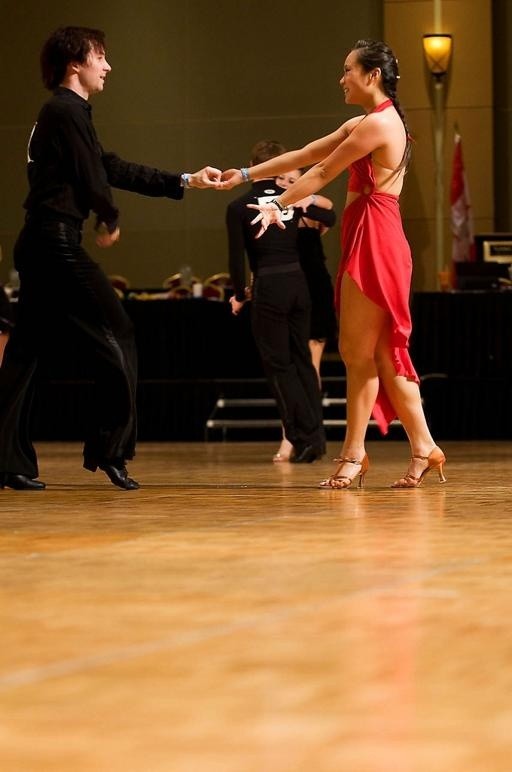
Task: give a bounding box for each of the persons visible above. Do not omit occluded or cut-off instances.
[226,140,337,463]
[0,26,222,492]
[269,167,333,463]
[215,39,446,489]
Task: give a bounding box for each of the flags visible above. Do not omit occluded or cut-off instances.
[448,132,475,289]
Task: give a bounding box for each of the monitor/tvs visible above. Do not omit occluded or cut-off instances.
[474,231,512,288]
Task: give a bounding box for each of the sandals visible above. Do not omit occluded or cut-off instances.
[272,436,293,463]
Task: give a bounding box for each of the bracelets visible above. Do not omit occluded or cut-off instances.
[270,199,284,212]
[240,167,249,182]
[181,173,193,188]
[311,194,315,205]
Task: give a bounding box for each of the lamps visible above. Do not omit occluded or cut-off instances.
[421,33,454,290]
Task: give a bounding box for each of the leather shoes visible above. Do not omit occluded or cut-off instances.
[291,442,326,464]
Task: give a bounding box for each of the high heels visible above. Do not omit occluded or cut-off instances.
[319,454,371,490]
[83,451,139,489]
[0,474,45,491]
[390,444,447,490]
